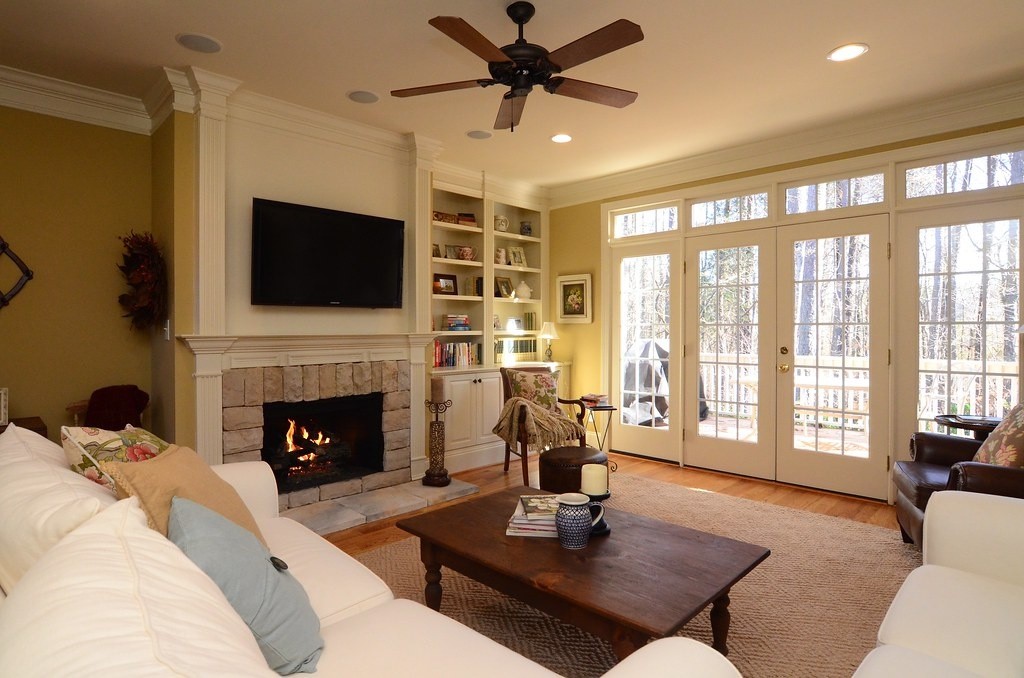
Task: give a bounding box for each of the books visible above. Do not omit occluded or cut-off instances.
[440,314,472,331]
[432,339,481,367]
[494,339,537,362]
[505,494,560,538]
[523,312,537,330]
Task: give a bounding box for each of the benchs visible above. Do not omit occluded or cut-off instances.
[739,373,932,442]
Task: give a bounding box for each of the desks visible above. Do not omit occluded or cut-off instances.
[0,416,47,440]
[395,485,771,663]
[580,399,617,471]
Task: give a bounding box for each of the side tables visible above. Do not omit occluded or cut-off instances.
[934,415,1003,441]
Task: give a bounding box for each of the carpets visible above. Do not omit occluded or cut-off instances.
[349,474,922,678]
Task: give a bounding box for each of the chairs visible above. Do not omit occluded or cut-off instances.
[500,367,585,486]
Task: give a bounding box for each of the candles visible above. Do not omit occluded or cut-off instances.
[431,378,444,403]
[581,464,607,495]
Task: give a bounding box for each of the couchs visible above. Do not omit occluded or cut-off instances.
[892,432,1024,550]
[851,492,1024,678]
[0,422,742,678]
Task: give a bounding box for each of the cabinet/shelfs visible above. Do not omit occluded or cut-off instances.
[431,173,544,449]
[556,273,592,324]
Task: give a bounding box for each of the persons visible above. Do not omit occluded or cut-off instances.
[501,283,510,295]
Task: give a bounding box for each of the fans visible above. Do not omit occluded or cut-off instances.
[391,1,644,129]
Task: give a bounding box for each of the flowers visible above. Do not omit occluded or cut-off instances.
[114,230,167,331]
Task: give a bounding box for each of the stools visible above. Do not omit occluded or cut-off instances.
[539,445,609,496]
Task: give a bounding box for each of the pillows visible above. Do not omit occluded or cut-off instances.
[972,403,1024,470]
[167,497,324,676]
[61,424,170,494]
[101,444,268,549]
[506,369,561,412]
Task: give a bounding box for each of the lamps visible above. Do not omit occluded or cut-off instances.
[537,322,560,362]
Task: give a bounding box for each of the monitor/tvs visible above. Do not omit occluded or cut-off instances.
[251,196,405,309]
